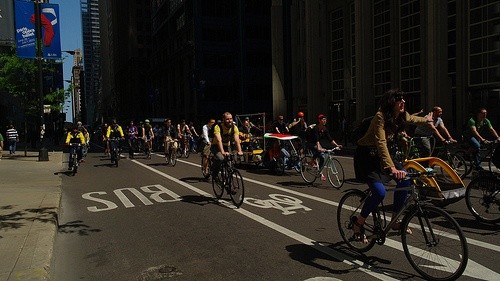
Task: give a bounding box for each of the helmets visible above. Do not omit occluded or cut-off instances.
[317,114,324,122]
[298,111,304,117]
[113,120,118,124]
[145,120,149,123]
[77,121,82,125]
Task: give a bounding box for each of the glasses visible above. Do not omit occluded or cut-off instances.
[395,94,410,101]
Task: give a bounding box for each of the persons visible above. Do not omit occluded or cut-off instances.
[6,125,19,154]
[0,134,4,159]
[400,105,500,172]
[210,112,242,193]
[65,112,341,180]
[352,89,433,242]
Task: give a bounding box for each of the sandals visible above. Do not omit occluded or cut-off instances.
[353,218,367,241]
[391,218,412,235]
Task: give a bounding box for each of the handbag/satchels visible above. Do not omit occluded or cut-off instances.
[356,144,381,170]
[16,136,19,142]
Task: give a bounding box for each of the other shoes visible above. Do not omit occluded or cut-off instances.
[321,177,327,181]
[309,161,317,167]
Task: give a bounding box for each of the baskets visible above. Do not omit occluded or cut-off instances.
[472,171,498,192]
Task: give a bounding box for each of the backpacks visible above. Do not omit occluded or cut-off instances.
[303,123,321,143]
[460,112,486,133]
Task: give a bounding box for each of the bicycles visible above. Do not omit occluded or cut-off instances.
[394,134,500,226]
[64,133,199,177]
[336,156,469,281]
[199,143,246,209]
[260,127,346,191]
